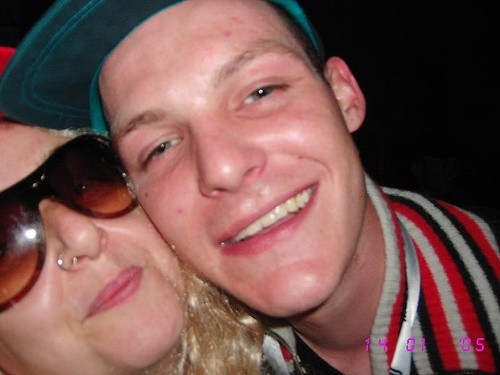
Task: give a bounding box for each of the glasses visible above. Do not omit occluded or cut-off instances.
[0,134,140,313]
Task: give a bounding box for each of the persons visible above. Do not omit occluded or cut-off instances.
[0,43,271,375]
[0,0,500,375]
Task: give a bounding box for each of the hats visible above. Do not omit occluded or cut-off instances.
[0,0,326,140]
